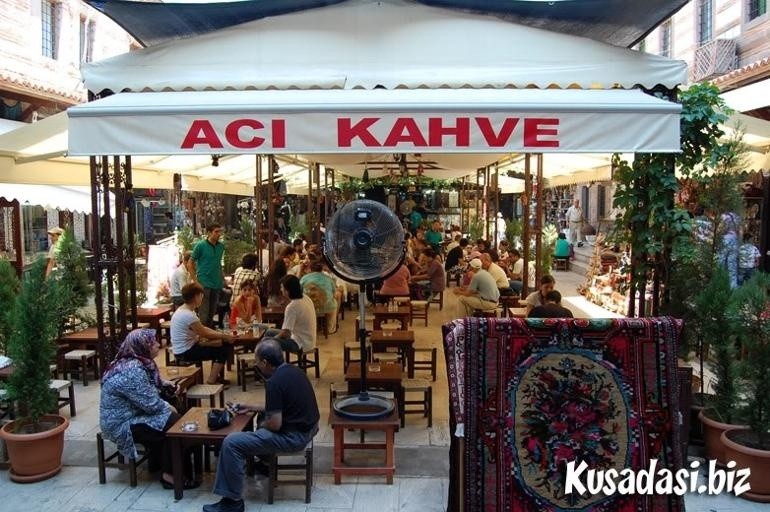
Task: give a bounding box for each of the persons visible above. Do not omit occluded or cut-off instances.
[202,339,320,511]
[169,200,586,390]
[44,227,66,280]
[164,211,179,234]
[99,329,202,490]
[717,204,743,290]
[737,233,762,286]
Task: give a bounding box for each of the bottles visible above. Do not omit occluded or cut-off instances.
[388,300,400,312]
[253,318,260,337]
[223,312,231,334]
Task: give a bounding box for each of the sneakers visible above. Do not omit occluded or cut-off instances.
[256,379,264,386]
[207,376,230,389]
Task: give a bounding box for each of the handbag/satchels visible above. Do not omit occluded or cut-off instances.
[208,409,230,427]
[583,223,596,235]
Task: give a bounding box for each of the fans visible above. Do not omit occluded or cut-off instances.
[322,200,405,421]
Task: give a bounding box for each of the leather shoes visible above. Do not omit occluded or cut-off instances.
[203,497,244,512]
[255,460,270,475]
[161,477,202,489]
[578,243,583,247]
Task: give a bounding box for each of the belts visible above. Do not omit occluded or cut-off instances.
[481,296,497,304]
[572,221,582,223]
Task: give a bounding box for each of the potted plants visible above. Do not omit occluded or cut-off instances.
[1,270,70,482]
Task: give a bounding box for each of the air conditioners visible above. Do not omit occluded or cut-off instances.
[693,38,739,84]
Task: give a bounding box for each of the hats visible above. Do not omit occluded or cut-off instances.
[471,251,481,258]
[468,259,482,269]
